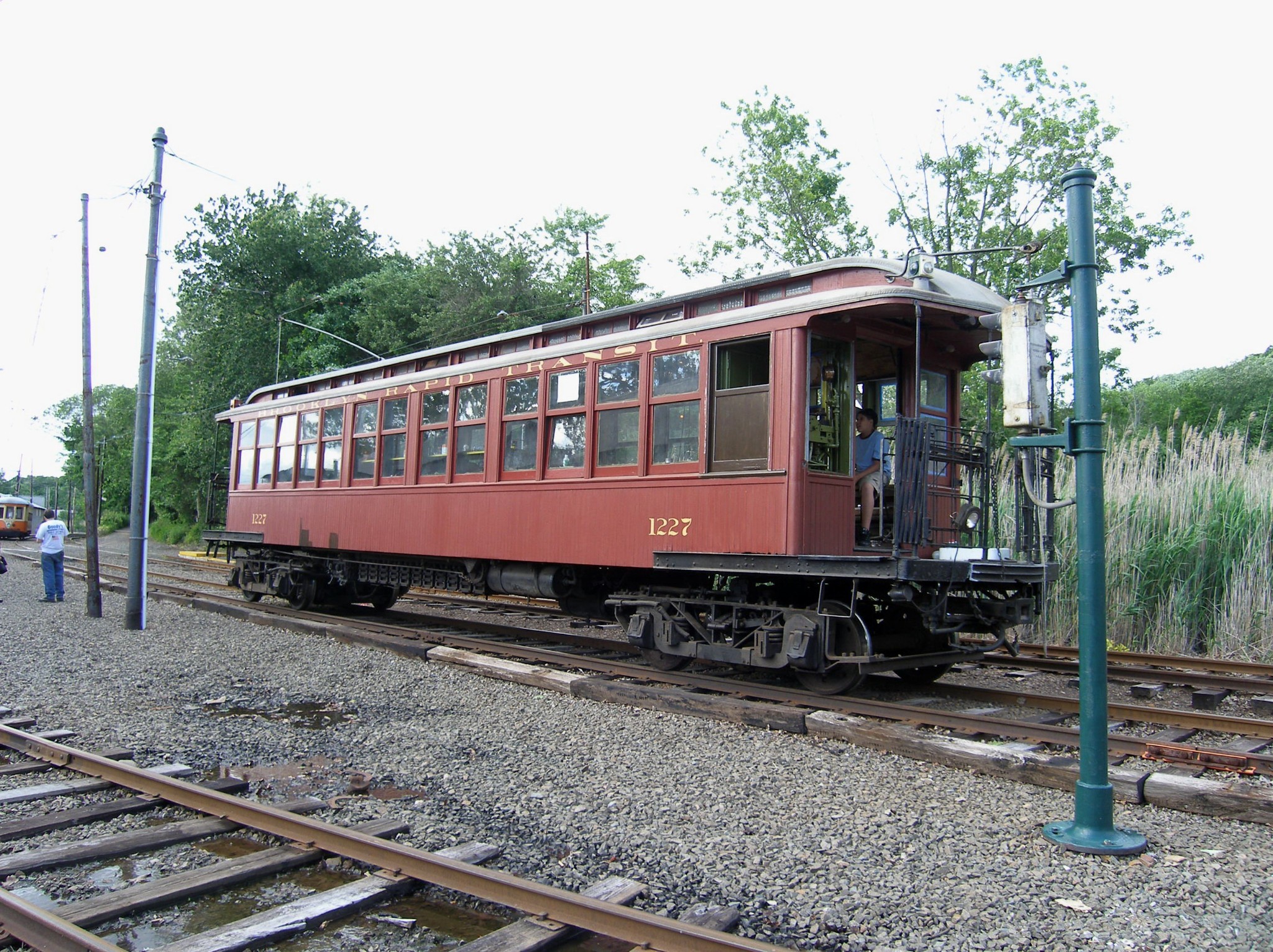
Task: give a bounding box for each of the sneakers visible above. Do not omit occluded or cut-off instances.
[857,528,870,546]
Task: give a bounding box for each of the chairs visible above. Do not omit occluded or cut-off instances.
[855,484,894,540]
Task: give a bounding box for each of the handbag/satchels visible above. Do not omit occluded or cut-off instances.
[0,561,7,574]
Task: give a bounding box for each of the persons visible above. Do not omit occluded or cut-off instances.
[8,507,13,519]
[855,408,892,546]
[35,509,69,603]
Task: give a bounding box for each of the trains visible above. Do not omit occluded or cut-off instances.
[0,496,33,540]
[198,250,1060,696]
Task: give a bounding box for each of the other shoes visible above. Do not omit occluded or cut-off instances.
[39,597,56,602]
[57,598,63,601]
[0,599,3,603]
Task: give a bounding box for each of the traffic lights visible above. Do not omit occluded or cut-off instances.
[979,301,1050,429]
